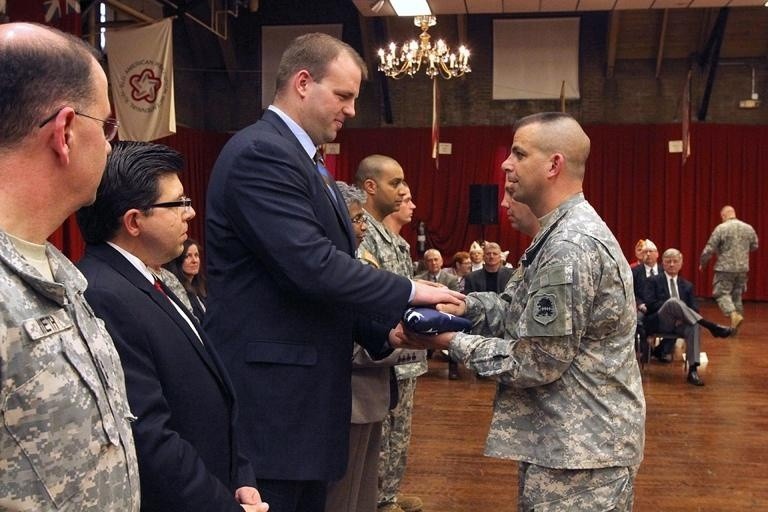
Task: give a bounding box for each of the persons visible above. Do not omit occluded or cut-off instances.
[631,240,676,364]
[630,239,644,268]
[394,111,646,511]
[442,250,471,293]
[644,247,734,386]
[200,33,466,512]
[0,22,143,512]
[415,249,459,292]
[72,141,269,512]
[469,241,485,271]
[699,205,758,337]
[169,238,206,315]
[325,179,428,512]
[377,182,423,512]
[500,173,541,293]
[465,243,514,295]
[353,154,413,279]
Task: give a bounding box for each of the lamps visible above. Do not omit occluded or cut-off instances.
[374,15,473,82]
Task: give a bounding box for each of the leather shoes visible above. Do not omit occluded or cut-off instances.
[712,324,734,339]
[687,370,705,386]
[394,489,423,512]
[448,371,461,380]
[378,503,404,512]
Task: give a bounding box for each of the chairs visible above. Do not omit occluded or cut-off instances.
[646,331,692,374]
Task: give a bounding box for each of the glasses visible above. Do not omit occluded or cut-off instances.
[130,193,193,213]
[37,107,122,142]
[349,214,370,225]
[461,262,472,265]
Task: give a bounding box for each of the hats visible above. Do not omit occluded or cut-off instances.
[468,241,483,255]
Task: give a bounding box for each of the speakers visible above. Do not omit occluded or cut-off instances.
[466,183,499,226]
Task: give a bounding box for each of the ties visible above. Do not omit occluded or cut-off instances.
[308,149,339,208]
[650,268,656,276]
[153,276,172,302]
[669,277,679,299]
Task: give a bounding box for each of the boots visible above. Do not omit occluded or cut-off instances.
[730,312,744,337]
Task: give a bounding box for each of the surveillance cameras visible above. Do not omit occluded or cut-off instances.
[738,99,761,110]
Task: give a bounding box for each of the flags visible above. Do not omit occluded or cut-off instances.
[431,79,440,170]
[104,20,176,140]
[681,81,692,165]
